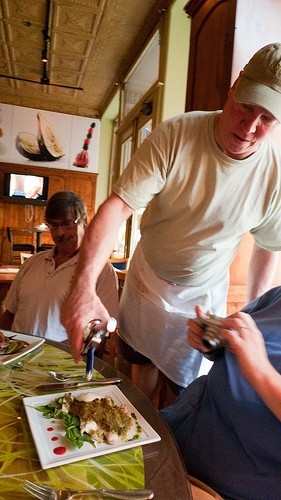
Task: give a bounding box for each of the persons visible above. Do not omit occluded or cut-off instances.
[0,190,118,346]
[159,285,281,500]
[61,42,280,409]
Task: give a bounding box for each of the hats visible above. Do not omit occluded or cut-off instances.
[234,42,281,123]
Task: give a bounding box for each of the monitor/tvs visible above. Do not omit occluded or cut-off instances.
[5,172,49,200]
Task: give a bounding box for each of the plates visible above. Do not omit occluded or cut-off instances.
[0,330,46,366]
[22,384,161,470]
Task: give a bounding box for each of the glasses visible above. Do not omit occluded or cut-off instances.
[44,215,82,229]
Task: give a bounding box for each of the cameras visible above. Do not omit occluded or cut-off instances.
[196,314,239,349]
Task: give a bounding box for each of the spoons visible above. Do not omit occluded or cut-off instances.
[48,370,121,381]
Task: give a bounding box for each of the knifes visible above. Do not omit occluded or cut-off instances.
[36,376,122,390]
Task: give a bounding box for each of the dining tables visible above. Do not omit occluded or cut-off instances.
[0,331,193,500]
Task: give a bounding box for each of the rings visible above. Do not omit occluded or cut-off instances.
[239,327,249,332]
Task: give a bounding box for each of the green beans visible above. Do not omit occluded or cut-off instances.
[5,342,23,354]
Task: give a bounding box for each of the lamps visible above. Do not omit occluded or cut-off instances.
[41,29,51,42]
[41,49,48,62]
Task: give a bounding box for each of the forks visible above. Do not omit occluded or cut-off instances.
[23,479,154,500]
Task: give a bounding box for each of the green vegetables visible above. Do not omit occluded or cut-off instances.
[35,396,97,449]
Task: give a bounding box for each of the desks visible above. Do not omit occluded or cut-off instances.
[17,228,50,253]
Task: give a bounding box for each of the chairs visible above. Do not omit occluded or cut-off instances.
[40,243,54,251]
[7,226,34,265]
